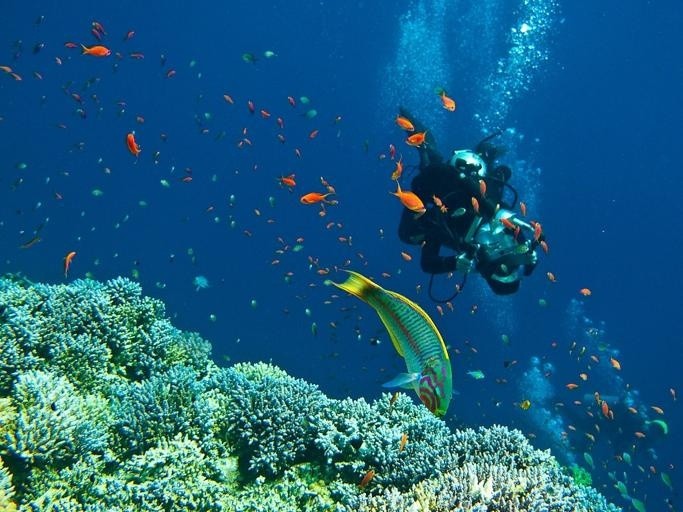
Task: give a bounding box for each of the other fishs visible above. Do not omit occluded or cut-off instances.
[1,16,683,511]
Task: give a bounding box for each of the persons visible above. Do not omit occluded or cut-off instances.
[398,104,511,272]
